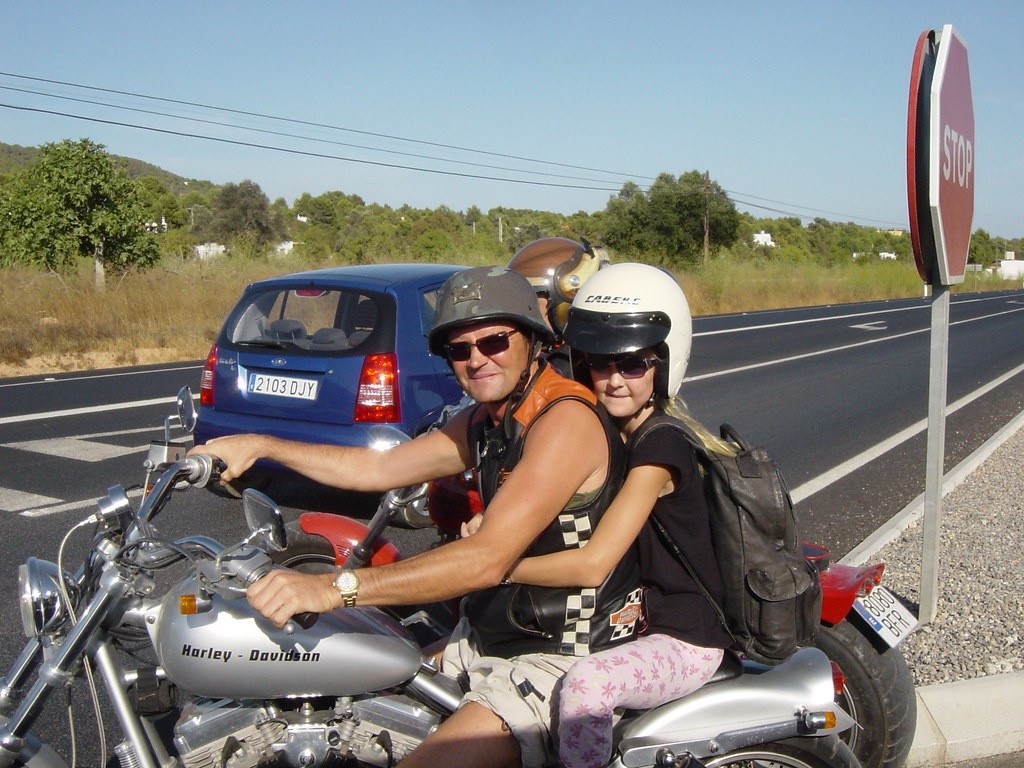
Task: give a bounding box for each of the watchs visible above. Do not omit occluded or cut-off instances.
[332,569,360,608]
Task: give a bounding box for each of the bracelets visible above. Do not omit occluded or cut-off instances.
[500,576,511,585]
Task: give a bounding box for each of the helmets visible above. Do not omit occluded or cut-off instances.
[508,237,610,337]
[563,262,693,400]
[428,264,556,359]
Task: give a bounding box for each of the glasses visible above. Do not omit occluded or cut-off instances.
[584,352,659,379]
[443,328,520,361]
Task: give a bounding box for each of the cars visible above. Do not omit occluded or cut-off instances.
[192,264,475,531]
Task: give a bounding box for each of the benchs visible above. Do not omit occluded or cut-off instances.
[255,298,379,353]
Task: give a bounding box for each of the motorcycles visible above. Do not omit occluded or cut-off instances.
[0,383,920,767]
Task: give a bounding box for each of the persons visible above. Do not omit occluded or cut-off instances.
[186,238,725,768]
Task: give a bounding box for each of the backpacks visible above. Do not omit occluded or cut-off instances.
[628,414,822,666]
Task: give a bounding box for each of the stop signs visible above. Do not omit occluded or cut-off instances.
[930,24,975,286]
